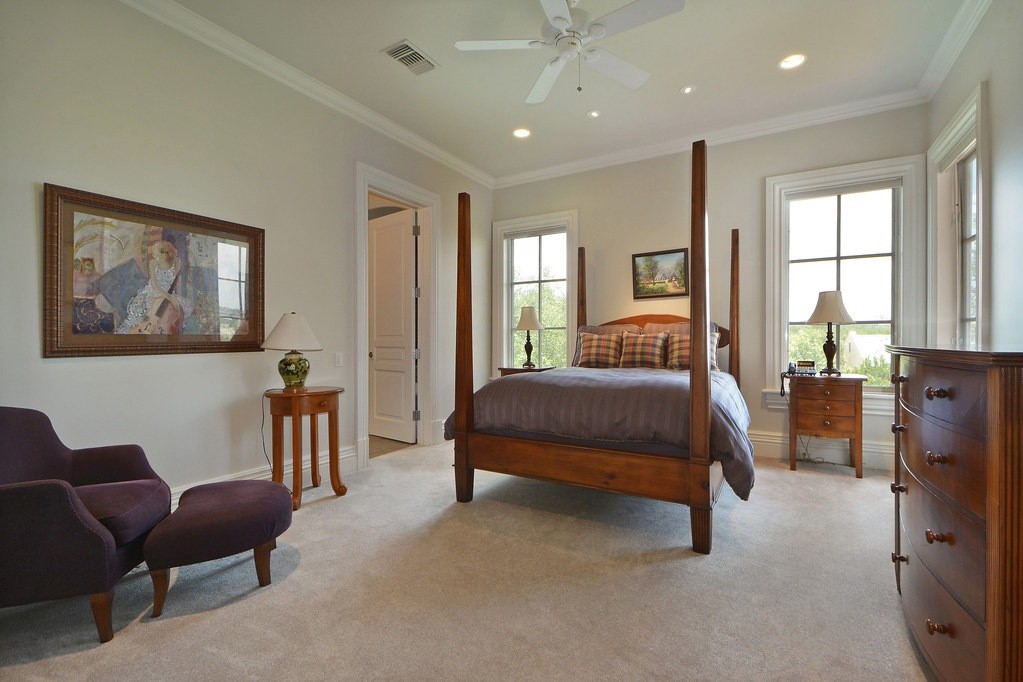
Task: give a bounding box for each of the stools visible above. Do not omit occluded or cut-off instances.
[142,480,293,619]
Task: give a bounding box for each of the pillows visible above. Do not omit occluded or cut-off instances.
[577,332,622,367]
[618,330,669,368]
[571,324,642,365]
[640,322,718,333]
[667,332,721,371]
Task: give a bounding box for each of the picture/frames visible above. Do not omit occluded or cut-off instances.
[632,248,689,299]
[44,182,266,357]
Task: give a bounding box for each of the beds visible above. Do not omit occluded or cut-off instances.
[443,139,755,553]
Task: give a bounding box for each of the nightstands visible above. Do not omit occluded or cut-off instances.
[497,366,555,377]
[784,372,869,479]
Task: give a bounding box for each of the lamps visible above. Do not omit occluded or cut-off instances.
[261,312,324,391]
[805,291,856,376]
[514,306,545,368]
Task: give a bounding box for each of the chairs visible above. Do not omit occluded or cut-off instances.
[0,405,171,643]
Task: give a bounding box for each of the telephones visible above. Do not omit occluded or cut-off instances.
[788,360,816,377]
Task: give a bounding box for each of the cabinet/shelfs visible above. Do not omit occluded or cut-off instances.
[886,344,1023,682]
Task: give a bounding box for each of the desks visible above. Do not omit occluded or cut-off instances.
[263,386,348,510]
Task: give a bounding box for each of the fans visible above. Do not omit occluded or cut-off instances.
[455,0,686,104]
